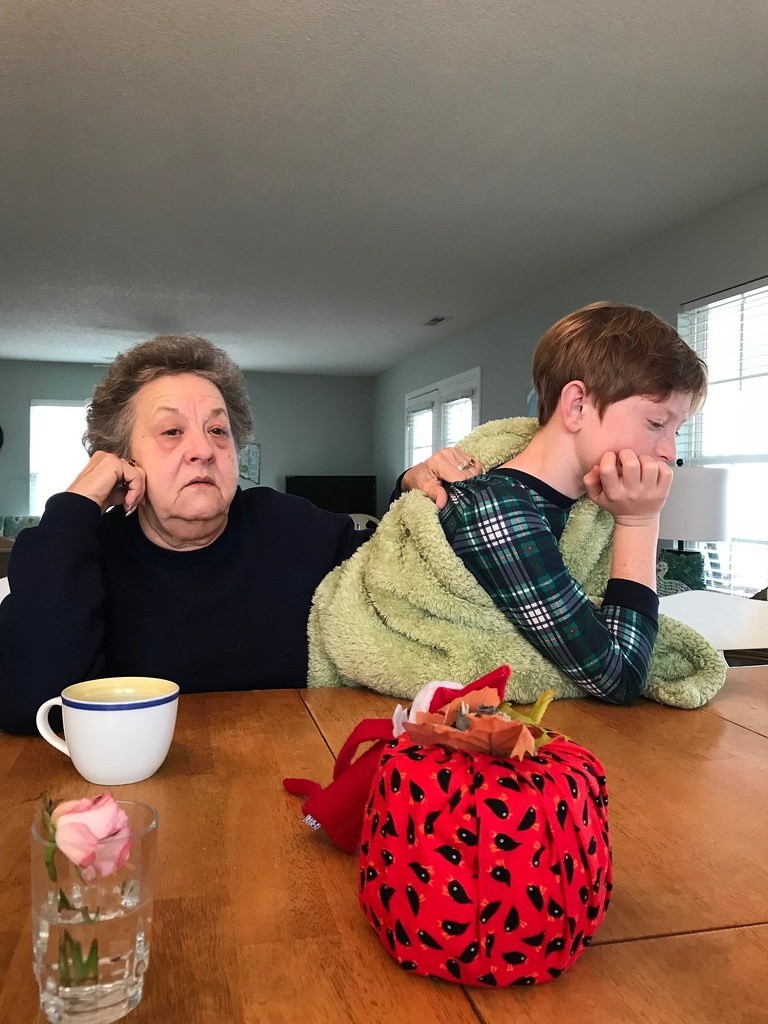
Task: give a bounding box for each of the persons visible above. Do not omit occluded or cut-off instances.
[439,301,707,708]
[0,335,481,740]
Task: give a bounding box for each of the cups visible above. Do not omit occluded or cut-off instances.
[36,677,180,786]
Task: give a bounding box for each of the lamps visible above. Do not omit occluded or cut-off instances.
[653,467,728,599]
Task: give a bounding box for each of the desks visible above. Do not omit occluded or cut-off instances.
[0,659,767,1023]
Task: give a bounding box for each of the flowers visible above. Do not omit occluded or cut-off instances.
[39,794,136,889]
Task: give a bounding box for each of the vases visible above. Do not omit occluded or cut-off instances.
[26,801,150,1024]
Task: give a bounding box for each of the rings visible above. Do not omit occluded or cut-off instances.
[457,462,469,471]
[468,458,476,468]
[128,458,137,467]
